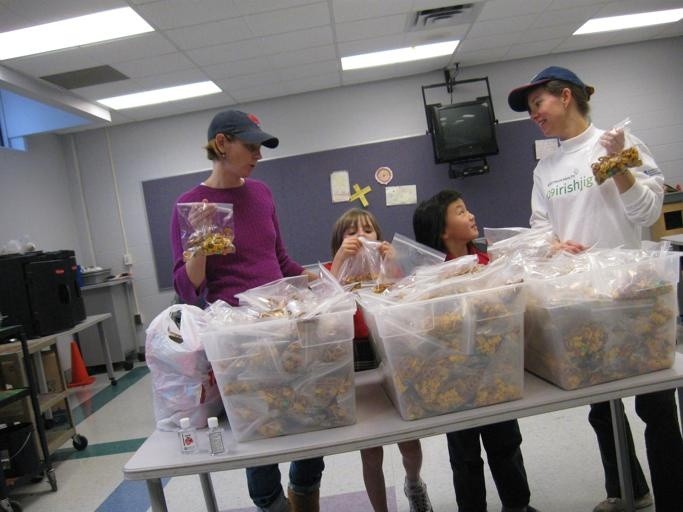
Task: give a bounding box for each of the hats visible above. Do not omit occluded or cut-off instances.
[508,66,590,112]
[207,110,279,148]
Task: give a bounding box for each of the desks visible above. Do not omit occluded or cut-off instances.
[76,279,135,367]
[121,350,683,512]
[56,312,113,382]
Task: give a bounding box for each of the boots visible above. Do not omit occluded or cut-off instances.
[287,486,320,512]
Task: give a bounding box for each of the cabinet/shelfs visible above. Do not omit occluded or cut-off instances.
[0,331,75,498]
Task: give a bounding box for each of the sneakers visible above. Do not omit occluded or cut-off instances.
[403,475,433,512]
[593,490,654,512]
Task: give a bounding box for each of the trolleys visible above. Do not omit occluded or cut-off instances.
[0,278,146,512]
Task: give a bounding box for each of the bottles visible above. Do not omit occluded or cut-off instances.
[177,417,197,453]
[206,416,226,456]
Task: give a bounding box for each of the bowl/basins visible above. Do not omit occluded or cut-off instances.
[80,268,111,287]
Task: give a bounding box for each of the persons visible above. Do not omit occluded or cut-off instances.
[412,189,544,512]
[169,106,326,511]
[316,204,437,512]
[504,63,681,512]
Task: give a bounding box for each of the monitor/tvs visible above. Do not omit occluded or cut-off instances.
[431,96,500,164]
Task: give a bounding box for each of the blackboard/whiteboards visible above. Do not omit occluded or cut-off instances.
[138,116,561,293]
